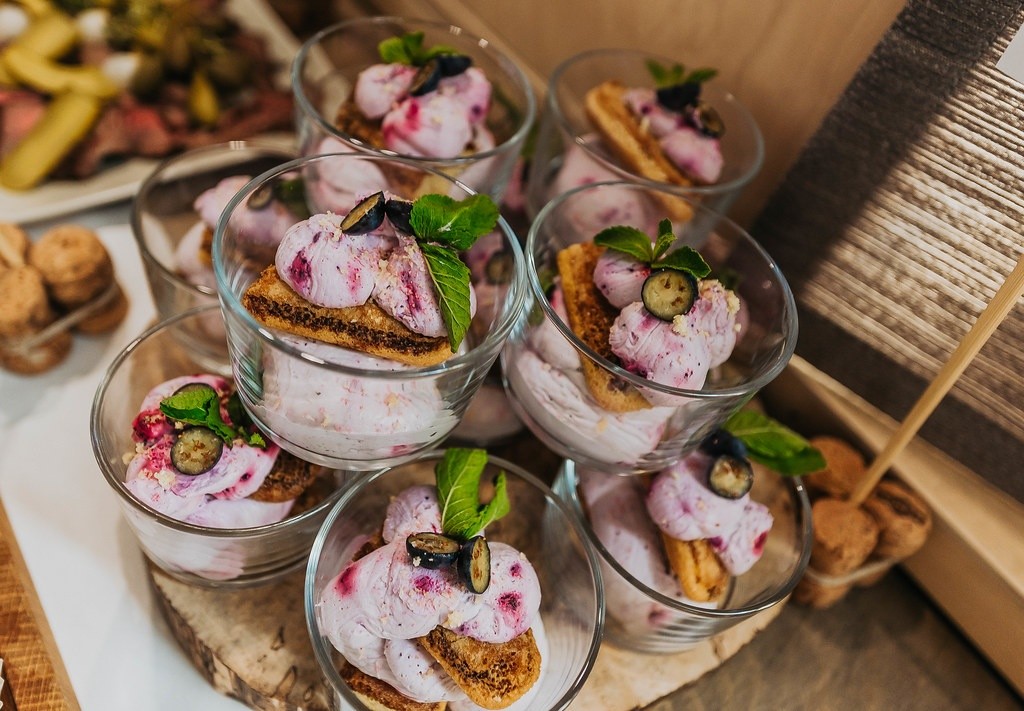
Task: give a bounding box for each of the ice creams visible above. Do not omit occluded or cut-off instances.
[111,27,829,711]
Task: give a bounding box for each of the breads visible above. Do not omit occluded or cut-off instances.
[798,438,933,608]
[0,222,128,376]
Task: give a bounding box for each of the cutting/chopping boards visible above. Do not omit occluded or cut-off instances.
[123,336,799,708]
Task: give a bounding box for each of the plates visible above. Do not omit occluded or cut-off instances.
[0,0,351,228]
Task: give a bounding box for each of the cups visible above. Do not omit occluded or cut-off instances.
[209,150,529,473]
[536,48,766,247]
[549,425,813,656]
[291,17,537,261]
[496,179,799,477]
[303,446,604,711]
[89,305,365,591]
[129,143,296,368]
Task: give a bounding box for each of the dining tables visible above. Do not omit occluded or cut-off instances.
[0,179,802,711]
